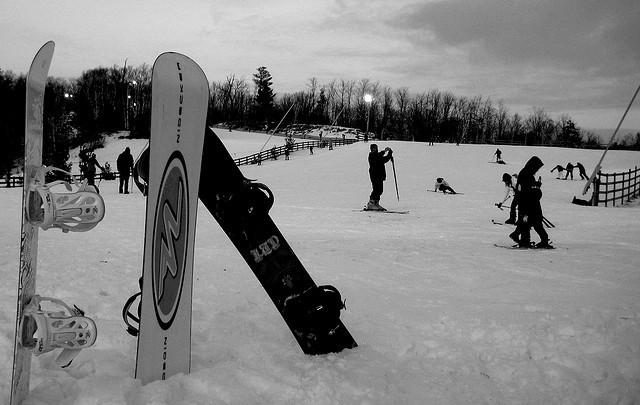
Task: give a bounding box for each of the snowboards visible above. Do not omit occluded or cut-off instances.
[8,40,105,405]
[122,52,210,385]
[199,126,358,354]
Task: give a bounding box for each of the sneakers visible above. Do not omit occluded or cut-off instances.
[443,190,447,193]
[374,202,384,210]
[509,232,520,243]
[119,190,123,193]
[367,201,380,210]
[124,191,130,194]
[451,191,456,194]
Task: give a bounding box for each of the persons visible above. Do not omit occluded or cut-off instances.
[498,173,521,224]
[429,139,433,146]
[523,188,549,249]
[456,136,461,146]
[309,140,333,155]
[573,162,590,180]
[100,161,116,181]
[366,144,393,210]
[494,148,502,162]
[85,153,103,185]
[256,146,290,165]
[435,177,457,194]
[509,155,544,249]
[551,165,566,180]
[117,147,134,193]
[565,163,573,180]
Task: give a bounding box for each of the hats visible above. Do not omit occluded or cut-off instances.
[501,173,512,181]
[92,153,96,157]
[125,147,131,151]
[437,178,442,182]
[370,144,377,150]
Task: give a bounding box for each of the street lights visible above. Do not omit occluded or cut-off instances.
[362,93,374,132]
[63,91,73,113]
[125,79,137,130]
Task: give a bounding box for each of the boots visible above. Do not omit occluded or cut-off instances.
[505,212,516,224]
[536,235,548,247]
